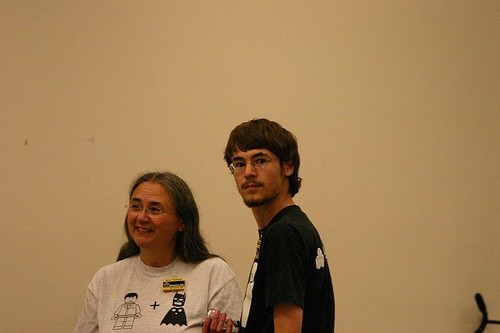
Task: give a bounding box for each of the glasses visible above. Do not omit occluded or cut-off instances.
[231,157,278,172]
[124,202,174,220]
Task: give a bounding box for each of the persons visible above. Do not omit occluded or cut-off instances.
[202,118,335,333]
[72,172,242,333]
